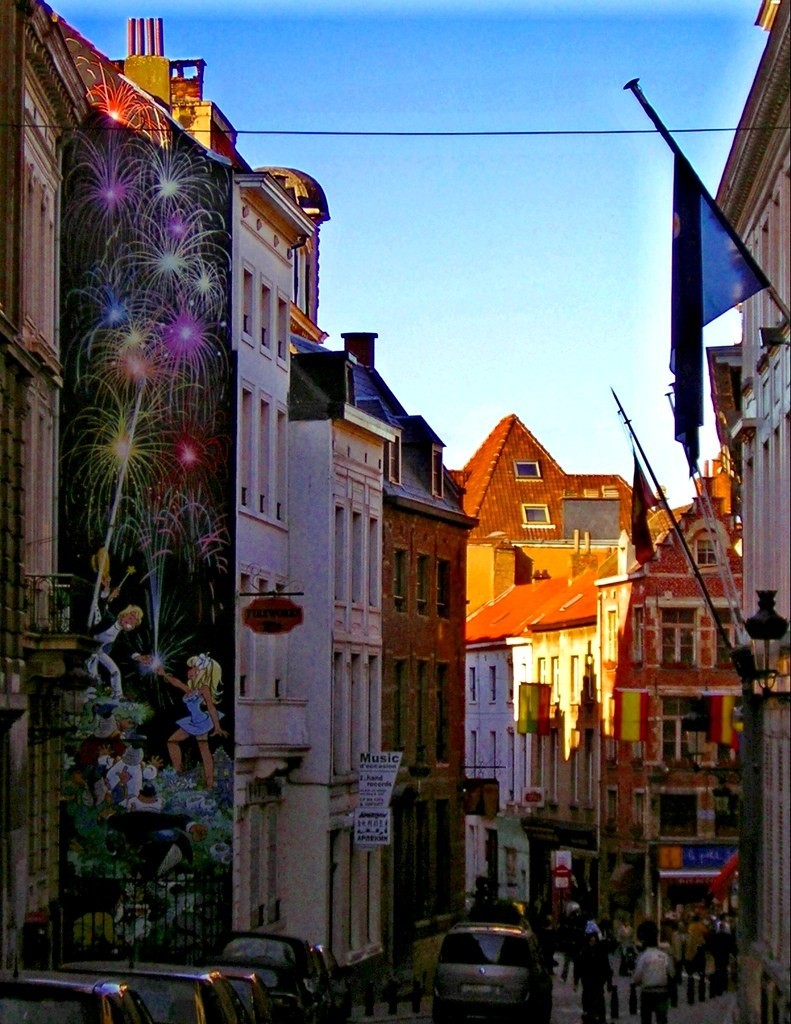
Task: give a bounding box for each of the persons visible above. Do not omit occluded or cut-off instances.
[472,877,738,1024]
[633,936,675,1024]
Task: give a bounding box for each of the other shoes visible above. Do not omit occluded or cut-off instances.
[572,986,577,992]
[688,1000,694,1006]
[671,1002,677,1008]
[699,996,705,1003]
[559,977,566,985]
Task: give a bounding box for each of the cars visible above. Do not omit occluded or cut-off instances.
[59,962,255,1024]
[430,920,556,1024]
[205,966,275,1024]
[216,954,315,1024]
[215,928,363,1024]
[0,969,161,1024]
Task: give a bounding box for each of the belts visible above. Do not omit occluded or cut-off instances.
[643,988,667,993]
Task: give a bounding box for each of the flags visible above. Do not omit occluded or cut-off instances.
[631,453,658,565]
[669,153,769,477]
